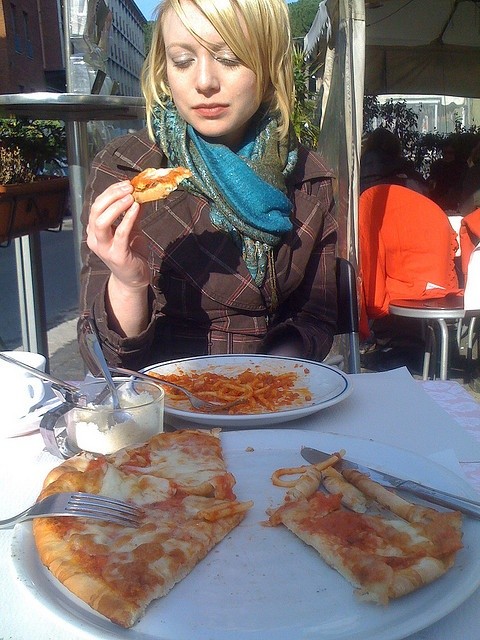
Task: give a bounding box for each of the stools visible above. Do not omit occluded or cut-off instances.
[384,241,480,382]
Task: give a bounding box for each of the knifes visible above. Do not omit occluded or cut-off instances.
[302,447,480,519]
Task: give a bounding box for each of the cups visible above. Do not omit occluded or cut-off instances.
[0,351,46,420]
[65,379,165,462]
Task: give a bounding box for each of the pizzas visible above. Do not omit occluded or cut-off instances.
[32,426,250,629]
[268,450,463,602]
[133,165,194,204]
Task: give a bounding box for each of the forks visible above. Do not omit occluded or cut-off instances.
[107,366,227,414]
[2,492,145,531]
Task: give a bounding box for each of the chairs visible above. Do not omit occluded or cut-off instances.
[337,258,362,375]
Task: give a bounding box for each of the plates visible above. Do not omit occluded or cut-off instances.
[11,430,480,639]
[2,379,64,438]
[129,353,356,429]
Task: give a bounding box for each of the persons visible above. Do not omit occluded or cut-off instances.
[361,127,430,352]
[459,147,480,214]
[425,136,466,200]
[77,0,339,378]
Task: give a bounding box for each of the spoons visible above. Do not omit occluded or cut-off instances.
[85,334,135,426]
